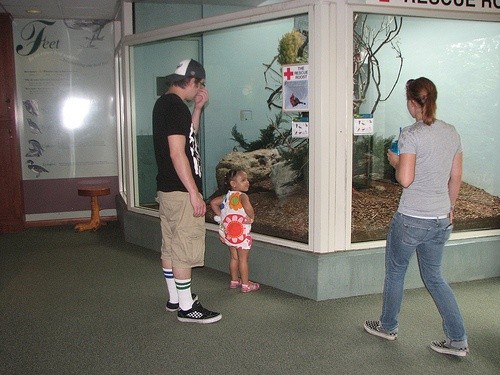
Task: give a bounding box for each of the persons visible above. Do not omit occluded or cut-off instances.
[210,168,260,292]
[362,77,470,356]
[153,58,222,323]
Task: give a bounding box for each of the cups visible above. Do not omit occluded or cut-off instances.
[392,139,400,157]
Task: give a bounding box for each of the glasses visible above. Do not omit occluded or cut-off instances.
[199,80,205,89]
[406,78,414,94]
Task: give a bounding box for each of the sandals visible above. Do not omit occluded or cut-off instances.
[241,281,260,292]
[230,278,242,288]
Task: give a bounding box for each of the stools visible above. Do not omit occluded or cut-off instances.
[73,187,110,232]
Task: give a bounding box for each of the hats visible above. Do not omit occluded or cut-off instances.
[167,59,205,82]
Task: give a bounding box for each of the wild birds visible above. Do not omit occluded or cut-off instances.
[23,99,50,178]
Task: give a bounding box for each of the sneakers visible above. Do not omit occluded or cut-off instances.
[364,320,397,340]
[165,293,198,312]
[177,300,221,323]
[430,339,466,357]
